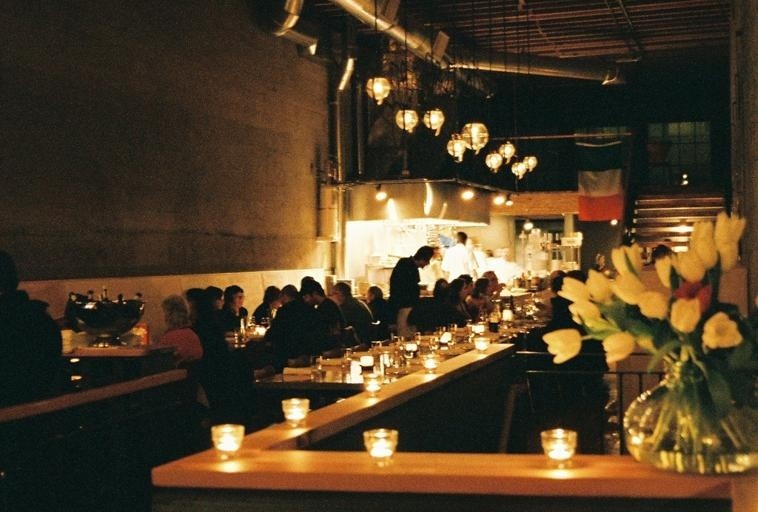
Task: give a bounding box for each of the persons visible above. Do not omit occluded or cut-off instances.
[2,251,63,404]
[430,231,503,328]
[158,285,248,367]
[654,244,674,257]
[543,269,587,332]
[254,275,391,373]
[388,246,434,317]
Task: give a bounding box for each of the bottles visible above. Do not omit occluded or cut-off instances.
[66,285,146,304]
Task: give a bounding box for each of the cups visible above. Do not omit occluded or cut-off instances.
[309,355,324,374]
[361,429,402,469]
[343,304,541,394]
[235,315,270,348]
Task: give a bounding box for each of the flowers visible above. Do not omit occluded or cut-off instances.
[543,206,758,458]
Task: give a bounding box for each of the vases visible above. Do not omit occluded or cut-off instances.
[620,359,757,479]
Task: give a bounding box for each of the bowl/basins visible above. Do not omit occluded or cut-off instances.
[65,301,145,350]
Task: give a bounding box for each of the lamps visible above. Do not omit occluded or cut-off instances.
[363,428,399,467]
[360,0,537,183]
